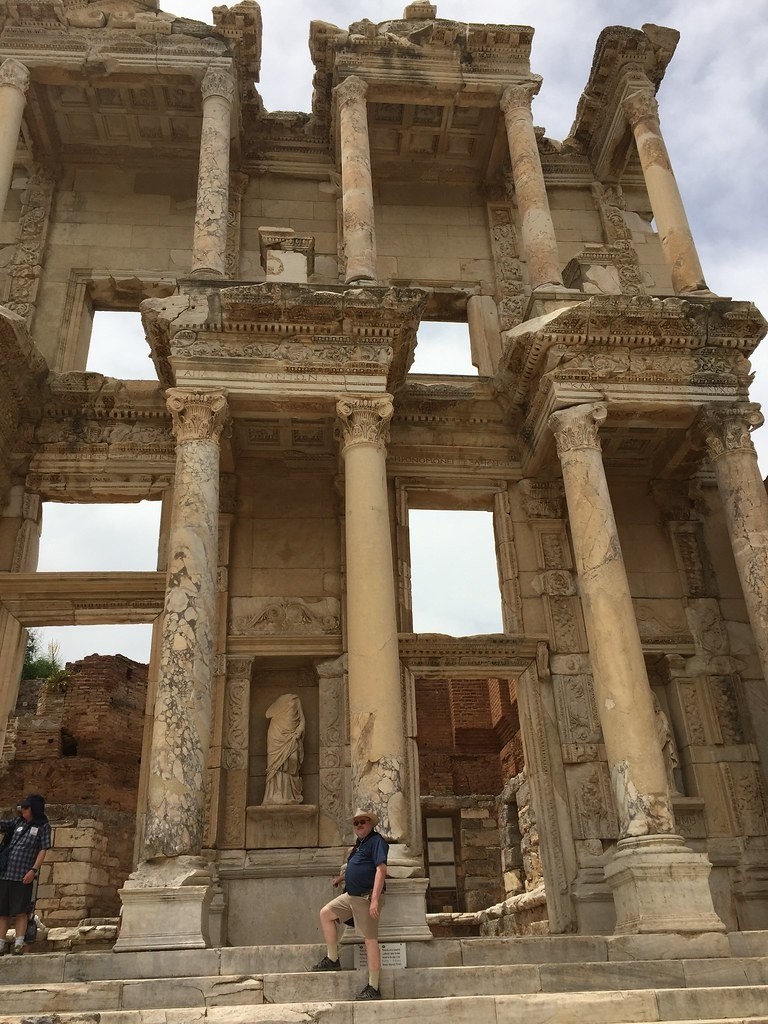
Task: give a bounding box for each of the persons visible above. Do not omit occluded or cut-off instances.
[0,794,51,958]
[311,808,389,1001]
[651,689,682,798]
[261,693,306,804]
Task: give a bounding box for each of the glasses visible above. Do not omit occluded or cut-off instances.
[353,819,370,826]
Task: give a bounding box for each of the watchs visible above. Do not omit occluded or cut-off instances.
[31,868,38,873]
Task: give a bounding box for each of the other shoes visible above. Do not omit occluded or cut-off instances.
[0,944,8,956]
[11,944,24,955]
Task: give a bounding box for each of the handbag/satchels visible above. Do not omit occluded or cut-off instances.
[343,888,354,927]
[0,843,12,872]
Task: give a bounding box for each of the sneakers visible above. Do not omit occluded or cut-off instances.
[356,983,381,1000]
[311,955,342,971]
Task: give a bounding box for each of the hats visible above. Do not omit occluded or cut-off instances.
[345,808,380,827]
[17,794,45,807]
